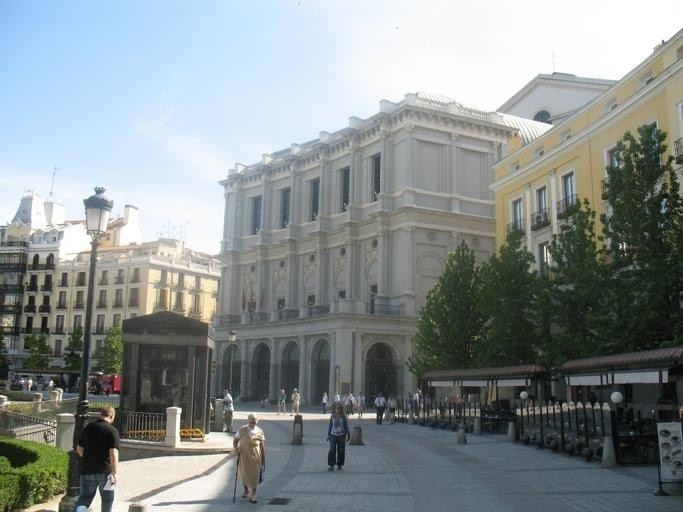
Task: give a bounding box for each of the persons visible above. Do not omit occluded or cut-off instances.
[221,388,233,432]
[326,403,350,470]
[290,388,300,416]
[321,387,430,425]
[15,376,55,391]
[72,406,120,511]
[277,388,286,413]
[588,392,597,402]
[232,413,265,503]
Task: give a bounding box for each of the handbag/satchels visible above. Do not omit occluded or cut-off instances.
[388,407,394,411]
[259,461,266,483]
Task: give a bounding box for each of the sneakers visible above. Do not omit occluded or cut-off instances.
[242,490,249,498]
[328,466,334,472]
[337,465,341,471]
[250,494,257,503]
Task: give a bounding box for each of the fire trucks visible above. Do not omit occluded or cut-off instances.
[86,370,120,396]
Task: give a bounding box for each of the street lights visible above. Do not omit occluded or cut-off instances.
[56,179,118,512]
[608,390,626,419]
[517,391,529,438]
[227,328,237,395]
